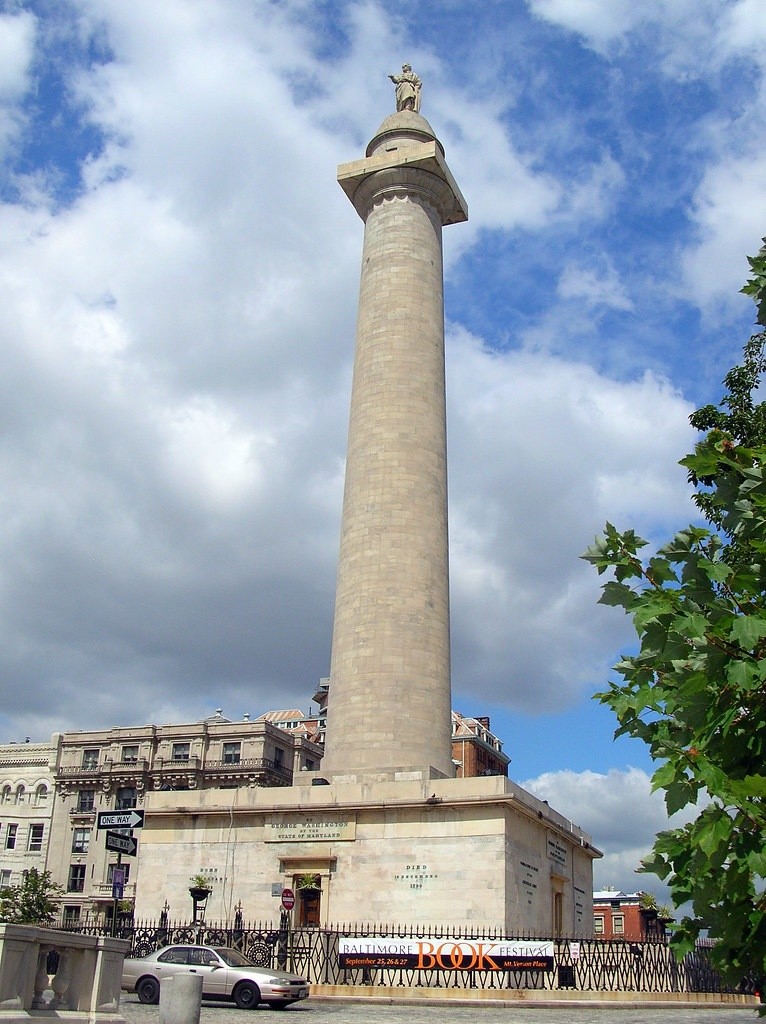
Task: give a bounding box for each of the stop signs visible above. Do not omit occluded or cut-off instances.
[280,888,296,911]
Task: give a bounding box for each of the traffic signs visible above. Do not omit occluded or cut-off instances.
[97,808,145,830]
[104,829,138,858]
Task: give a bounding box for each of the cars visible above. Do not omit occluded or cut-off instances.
[120,943,310,1010]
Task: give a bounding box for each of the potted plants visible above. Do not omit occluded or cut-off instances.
[297,872,322,902]
[190,874,211,901]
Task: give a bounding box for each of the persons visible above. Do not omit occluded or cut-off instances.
[387,63,423,112]
